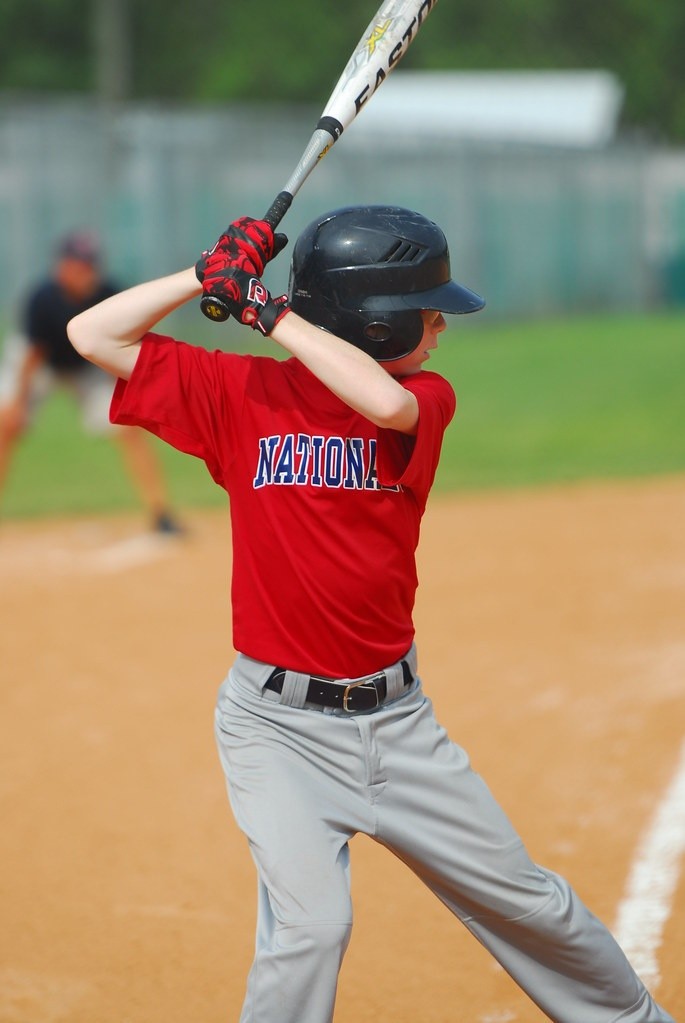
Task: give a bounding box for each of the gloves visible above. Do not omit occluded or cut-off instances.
[204,247,293,339]
[194,218,290,285]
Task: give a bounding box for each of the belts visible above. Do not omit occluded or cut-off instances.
[234,653,420,712]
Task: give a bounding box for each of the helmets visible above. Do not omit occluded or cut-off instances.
[287,208,487,364]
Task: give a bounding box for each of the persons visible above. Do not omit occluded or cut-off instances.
[0,229,204,544]
[60,202,676,1023]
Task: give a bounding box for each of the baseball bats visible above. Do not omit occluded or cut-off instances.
[199,0,433,320]
